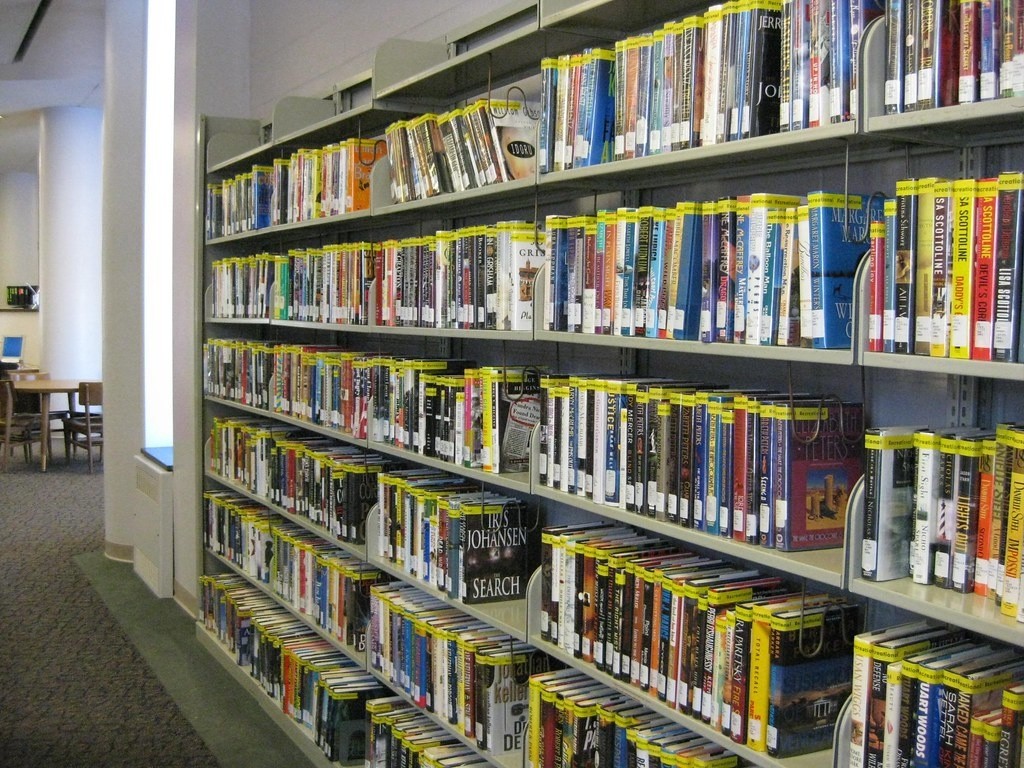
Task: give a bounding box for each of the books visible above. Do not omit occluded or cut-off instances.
[199,0,1023,768]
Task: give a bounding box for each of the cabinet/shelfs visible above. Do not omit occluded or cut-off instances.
[193,0,1024,768]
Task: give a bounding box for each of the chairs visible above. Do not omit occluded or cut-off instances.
[62,382,103,471]
[1,371,51,469]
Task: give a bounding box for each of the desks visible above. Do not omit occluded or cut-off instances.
[12,380,81,463]
